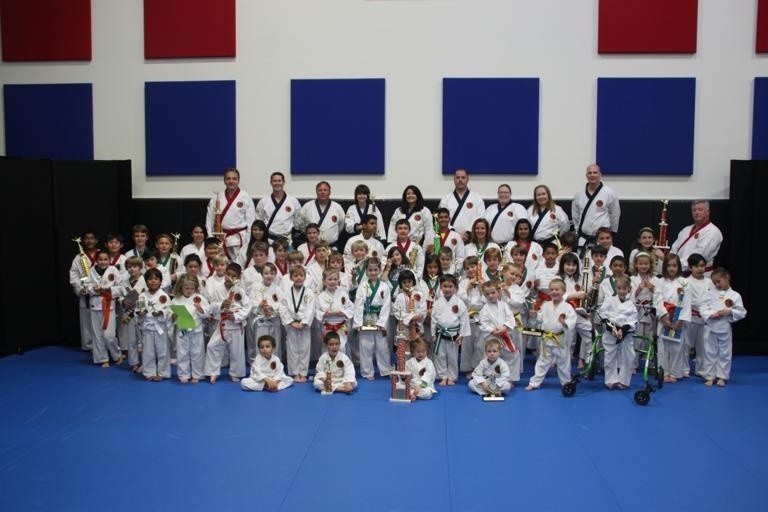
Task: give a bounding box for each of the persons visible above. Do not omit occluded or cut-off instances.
[70,165,747,402]
